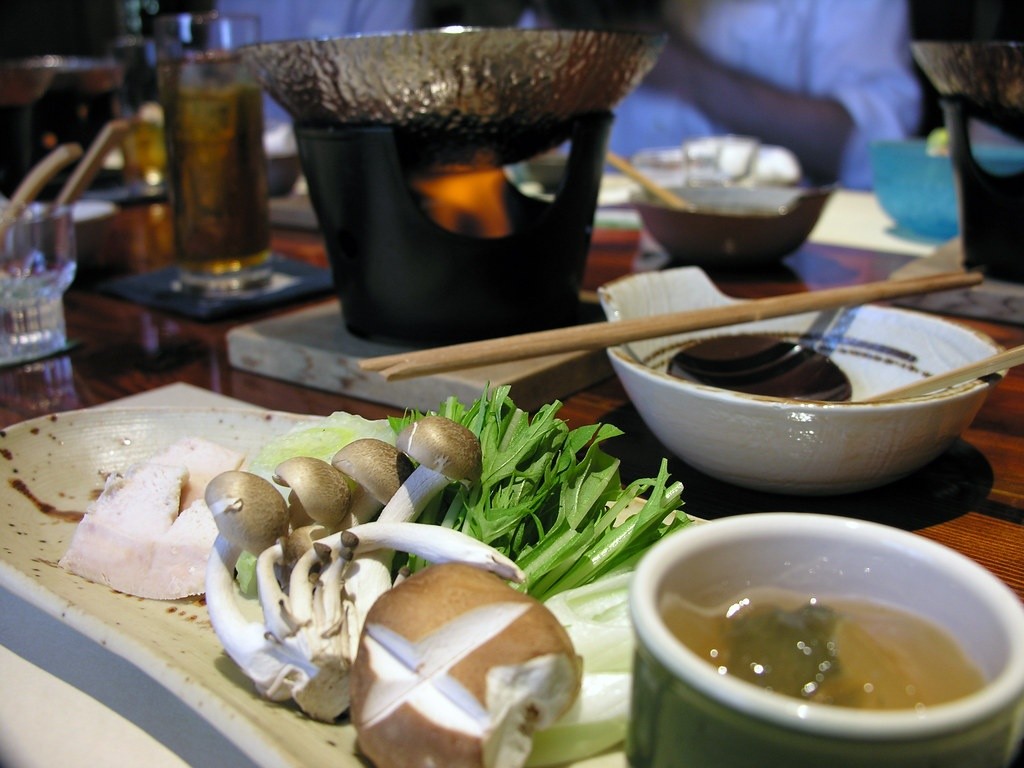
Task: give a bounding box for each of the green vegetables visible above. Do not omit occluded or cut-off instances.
[388,379,697,609]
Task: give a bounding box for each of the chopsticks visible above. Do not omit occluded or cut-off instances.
[356,268,985,382]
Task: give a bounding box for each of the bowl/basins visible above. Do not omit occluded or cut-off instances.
[629,183,836,268]
[604,301,1012,496]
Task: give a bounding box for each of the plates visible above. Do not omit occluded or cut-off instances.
[0,405,711,768]
[0,54,128,104]
[904,41,1024,107]
[236,24,671,126]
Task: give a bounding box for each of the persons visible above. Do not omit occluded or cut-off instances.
[205,0,920,193]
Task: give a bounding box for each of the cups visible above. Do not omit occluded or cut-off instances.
[626,511,1024,768]
[156,12,274,300]
[0,198,79,362]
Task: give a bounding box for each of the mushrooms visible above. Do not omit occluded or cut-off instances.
[201,417,636,768]
